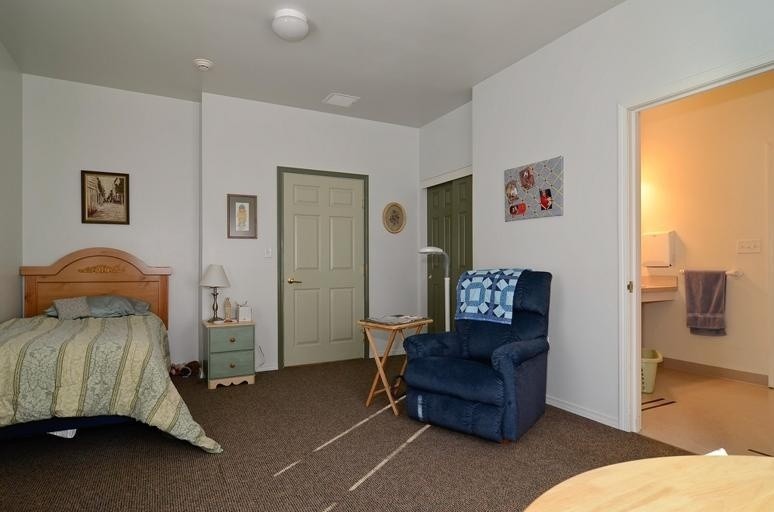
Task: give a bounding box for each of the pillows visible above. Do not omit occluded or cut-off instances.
[44,295,151,320]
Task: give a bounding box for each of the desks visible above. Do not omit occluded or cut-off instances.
[521,452,774,510]
[356,313,435,418]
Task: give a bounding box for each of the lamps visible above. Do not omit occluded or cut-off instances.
[198,263,232,323]
[417,245,454,332]
[270,7,311,42]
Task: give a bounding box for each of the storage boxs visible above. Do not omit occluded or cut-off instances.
[236,305,252,322]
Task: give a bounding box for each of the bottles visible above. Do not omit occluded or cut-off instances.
[223,296,230,319]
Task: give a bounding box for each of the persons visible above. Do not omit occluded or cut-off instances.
[540,190,551,210]
[510,181,518,201]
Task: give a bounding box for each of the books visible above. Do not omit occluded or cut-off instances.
[367,314,425,325]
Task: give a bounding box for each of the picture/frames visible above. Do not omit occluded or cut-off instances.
[80,169,131,226]
[226,193,259,240]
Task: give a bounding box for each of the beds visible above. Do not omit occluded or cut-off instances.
[0,246,225,456]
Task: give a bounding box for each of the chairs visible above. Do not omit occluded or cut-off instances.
[400,267,553,445]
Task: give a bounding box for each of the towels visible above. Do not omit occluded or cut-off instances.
[683,267,729,338]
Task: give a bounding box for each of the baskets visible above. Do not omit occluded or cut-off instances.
[641,348,663,394]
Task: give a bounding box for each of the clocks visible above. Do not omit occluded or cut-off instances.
[381,201,407,234]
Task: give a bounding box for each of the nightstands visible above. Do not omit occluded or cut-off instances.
[201,320,257,391]
